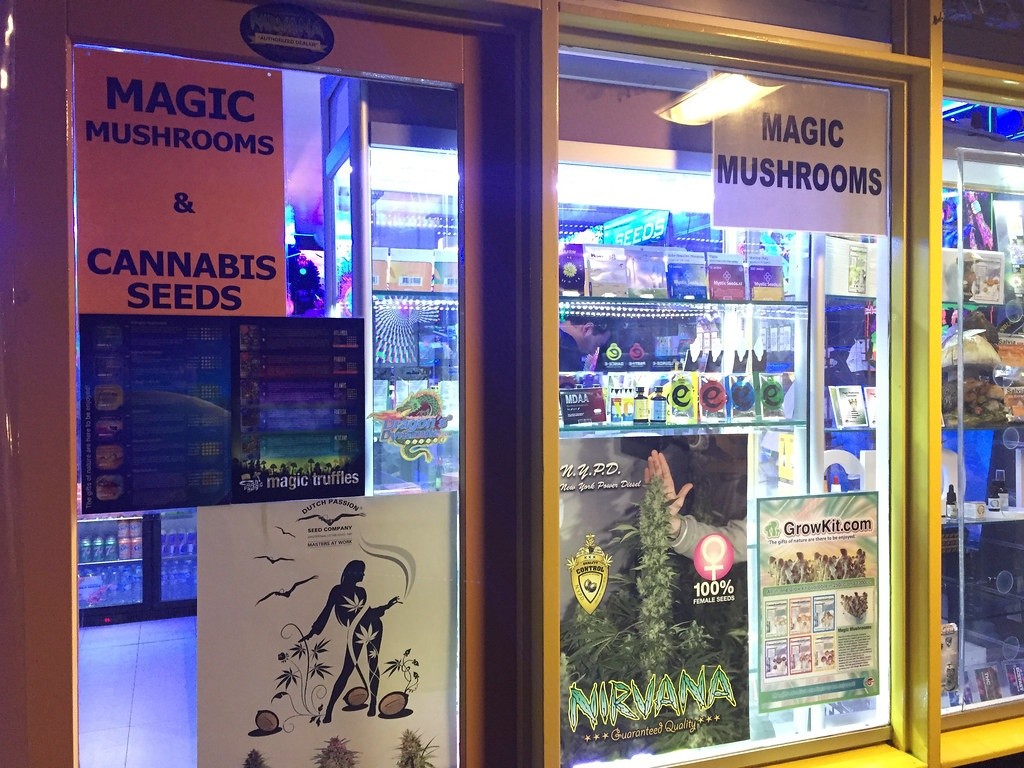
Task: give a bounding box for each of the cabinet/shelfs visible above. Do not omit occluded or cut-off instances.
[812,182,1024,719]
[321,73,814,677]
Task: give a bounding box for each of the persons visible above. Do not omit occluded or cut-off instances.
[645,449,747,563]
[559,316,612,372]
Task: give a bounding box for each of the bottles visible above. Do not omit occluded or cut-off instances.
[987,487,1002,511]
[847,244,869,295]
[650,387,669,423]
[161,526,196,555]
[993,469,1009,510]
[162,558,197,600]
[78,563,143,606]
[945,484,958,517]
[634,387,650,423]
